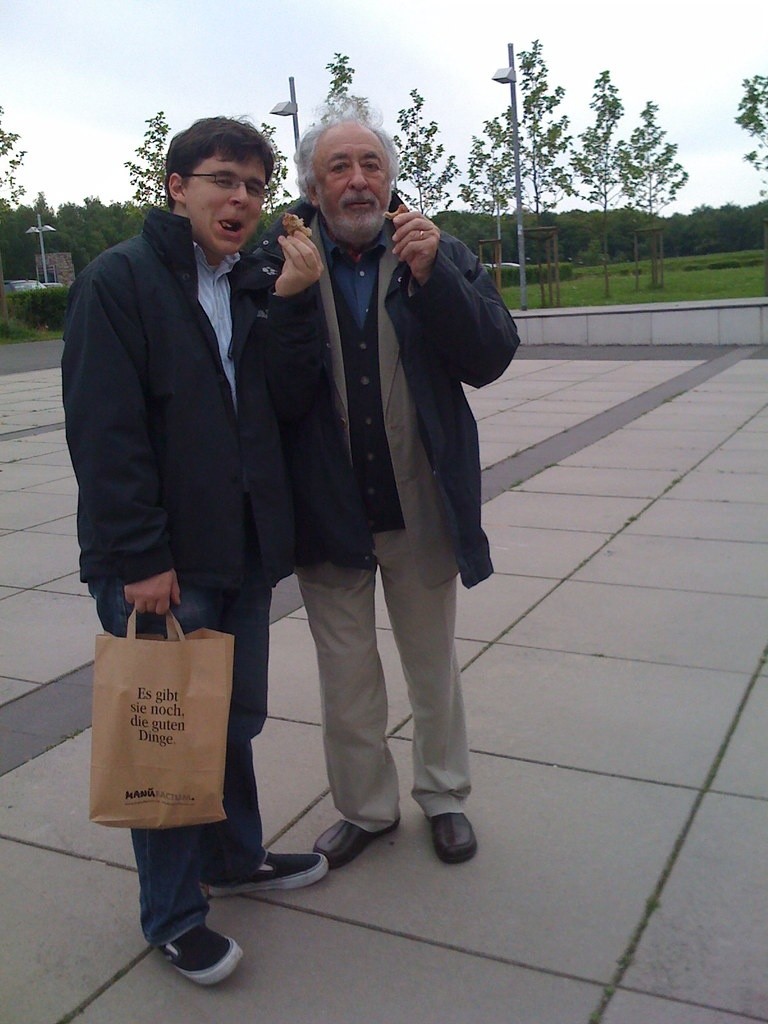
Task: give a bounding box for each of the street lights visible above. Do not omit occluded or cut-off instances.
[490,43,531,310]
[266,76,300,149]
[24,214,57,284]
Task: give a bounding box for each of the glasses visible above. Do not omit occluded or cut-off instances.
[182,171,270,197]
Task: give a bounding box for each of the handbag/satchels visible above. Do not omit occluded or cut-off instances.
[88,605,234,828]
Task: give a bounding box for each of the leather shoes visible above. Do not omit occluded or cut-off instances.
[429,812,477,863]
[313,817,403,868]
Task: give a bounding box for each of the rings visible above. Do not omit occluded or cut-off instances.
[420,231,424,239]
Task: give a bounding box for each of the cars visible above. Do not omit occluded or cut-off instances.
[4,279,47,294]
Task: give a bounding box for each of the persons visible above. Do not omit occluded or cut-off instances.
[61,115,330,987]
[252,115,520,870]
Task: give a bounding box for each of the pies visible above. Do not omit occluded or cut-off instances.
[384,204,407,219]
[282,212,312,237]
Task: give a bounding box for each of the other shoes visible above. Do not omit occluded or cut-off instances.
[207,851,329,895]
[159,918,242,984]
